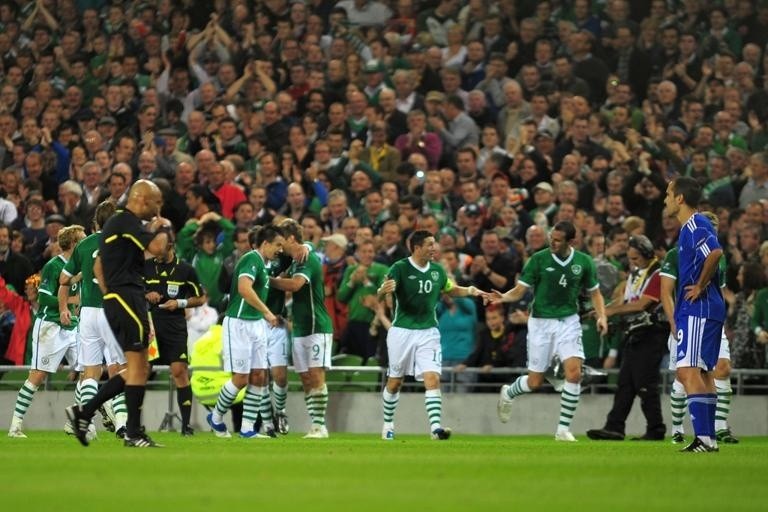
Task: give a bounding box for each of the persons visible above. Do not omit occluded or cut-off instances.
[0,196,17,224]
[207,225,282,439]
[175,212,237,314]
[339,218,360,242]
[1,170,21,199]
[0,224,33,365]
[65,178,171,447]
[374,223,410,265]
[485,222,606,443]
[186,303,219,367]
[750,240,768,393]
[178,184,221,232]
[469,231,522,292]
[10,230,26,254]
[660,211,740,446]
[337,226,389,286]
[456,300,534,394]
[58,180,97,236]
[586,236,673,440]
[286,182,320,245]
[230,202,255,230]
[323,234,348,357]
[190,315,263,434]
[58,283,73,328]
[380,182,399,220]
[521,225,552,264]
[0,269,67,391]
[437,247,462,283]
[19,178,43,198]
[59,202,129,443]
[737,156,768,217]
[664,177,724,454]
[206,164,248,215]
[1,4,768,176]
[395,172,664,230]
[101,173,130,208]
[8,225,92,439]
[28,214,66,270]
[356,193,392,225]
[326,189,352,232]
[338,240,391,365]
[268,219,335,438]
[249,225,316,440]
[716,200,768,254]
[299,213,319,241]
[377,231,489,440]
[350,171,372,207]
[432,275,476,392]
[134,230,207,436]
[360,289,416,391]
[218,226,251,294]
[10,199,50,245]
[249,185,274,227]
[720,262,763,395]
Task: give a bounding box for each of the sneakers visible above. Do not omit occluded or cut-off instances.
[498,385,513,423]
[264,428,276,437]
[555,433,577,443]
[429,427,452,440]
[679,439,713,452]
[304,427,329,438]
[718,433,736,443]
[587,428,626,439]
[64,424,75,434]
[632,434,663,440]
[8,430,27,437]
[381,423,395,439]
[240,431,269,438]
[66,405,88,446]
[207,413,232,437]
[672,433,683,443]
[123,436,163,447]
[276,412,289,432]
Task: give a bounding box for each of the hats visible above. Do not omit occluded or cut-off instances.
[426,90,441,103]
[531,181,554,192]
[323,236,349,248]
